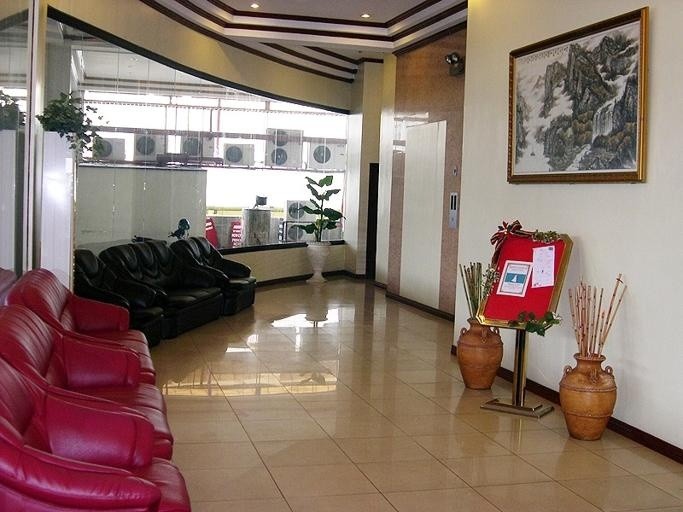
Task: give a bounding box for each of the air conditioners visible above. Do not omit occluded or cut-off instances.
[93,127,347,170]
[286,200,317,243]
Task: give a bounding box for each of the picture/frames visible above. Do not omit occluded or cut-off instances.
[509,11,649,183]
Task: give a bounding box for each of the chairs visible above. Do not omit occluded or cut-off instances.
[71,233,259,348]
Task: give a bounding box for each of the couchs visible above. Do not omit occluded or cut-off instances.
[1,268,156,385]
[1,359,191,512]
[1,303,174,459]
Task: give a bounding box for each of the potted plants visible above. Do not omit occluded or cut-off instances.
[304,176,344,282]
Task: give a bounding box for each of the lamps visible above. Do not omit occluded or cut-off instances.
[445,53,464,76]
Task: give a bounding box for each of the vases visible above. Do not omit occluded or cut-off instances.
[457,319,502,391]
[559,353,615,441]
